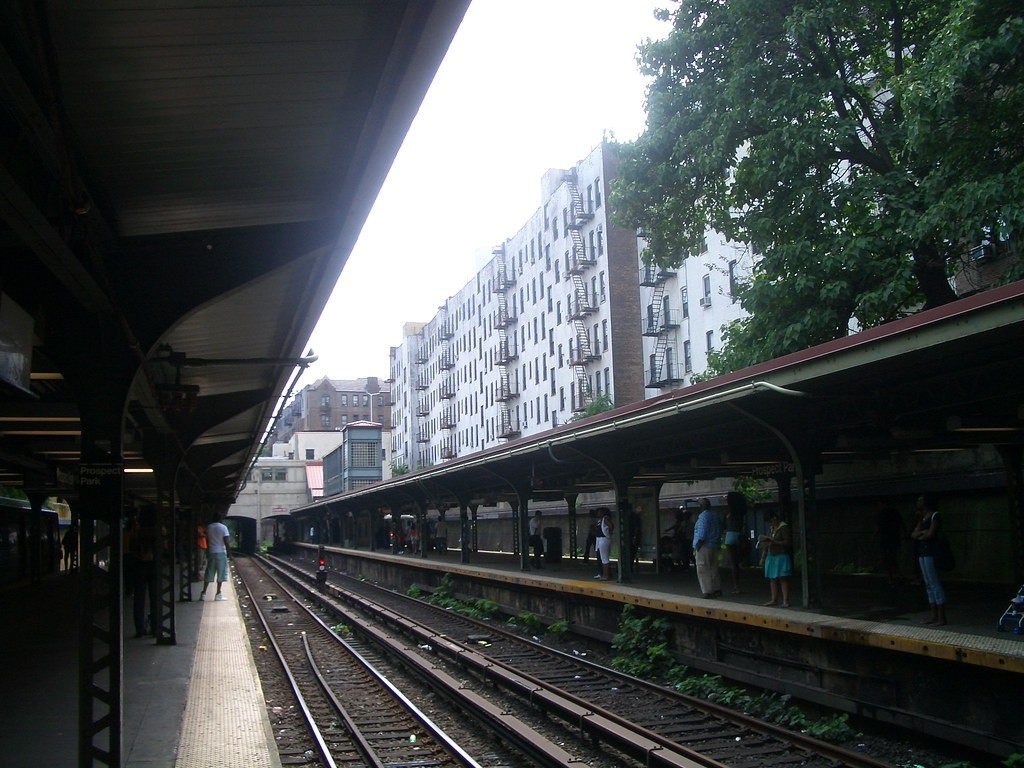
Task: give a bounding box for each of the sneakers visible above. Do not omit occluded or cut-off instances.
[199,591,206,601]
[215,591,221,600]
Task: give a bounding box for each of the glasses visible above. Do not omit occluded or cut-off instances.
[918,497,923,501]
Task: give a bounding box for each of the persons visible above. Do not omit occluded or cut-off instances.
[383,515,449,555]
[867,491,947,628]
[582,498,641,581]
[62,504,235,639]
[665,499,793,609]
[529,511,544,570]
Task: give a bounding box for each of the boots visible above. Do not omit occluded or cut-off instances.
[920,604,938,624]
[928,605,944,626]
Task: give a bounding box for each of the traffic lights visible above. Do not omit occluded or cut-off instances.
[319,546,324,571]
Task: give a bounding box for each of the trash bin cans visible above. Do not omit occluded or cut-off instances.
[662,536,673,554]
[547,538,563,558]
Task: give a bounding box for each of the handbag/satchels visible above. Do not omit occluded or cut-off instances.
[527,537,535,547]
[931,511,955,578]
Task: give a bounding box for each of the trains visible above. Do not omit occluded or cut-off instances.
[1,497,62,584]
[384,514,418,546]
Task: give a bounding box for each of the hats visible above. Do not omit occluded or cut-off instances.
[436,516,443,519]
[876,496,890,503]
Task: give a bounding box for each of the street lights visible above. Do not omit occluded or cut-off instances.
[364,389,380,421]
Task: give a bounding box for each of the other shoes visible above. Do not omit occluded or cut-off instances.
[599,576,611,581]
[761,601,778,606]
[530,559,542,569]
[781,602,791,608]
[593,574,601,579]
[414,551,416,555]
[136,629,147,637]
[701,590,721,599]
[410,552,413,554]
